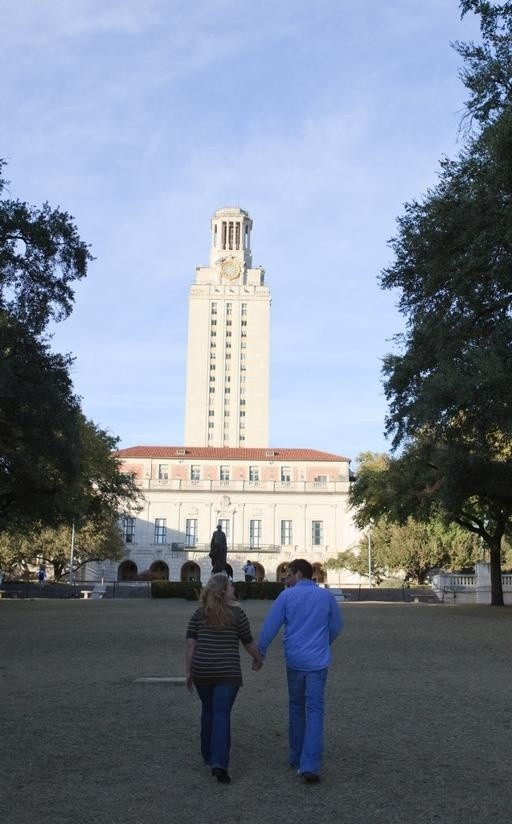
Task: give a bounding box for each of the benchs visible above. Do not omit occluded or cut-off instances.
[80,591,107,600]
[410,595,438,603]
[0,589,22,598]
[334,593,353,601]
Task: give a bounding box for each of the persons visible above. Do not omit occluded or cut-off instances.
[208,524,229,573]
[242,558,256,582]
[36,567,48,593]
[183,571,265,784]
[251,556,345,785]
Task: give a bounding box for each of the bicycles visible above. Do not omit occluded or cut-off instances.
[37,576,46,593]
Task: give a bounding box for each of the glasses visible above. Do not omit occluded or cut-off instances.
[286,571,297,577]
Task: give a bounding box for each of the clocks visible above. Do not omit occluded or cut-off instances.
[222,261,241,280]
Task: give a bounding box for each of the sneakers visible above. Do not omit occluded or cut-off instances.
[303,772,318,782]
[213,768,231,783]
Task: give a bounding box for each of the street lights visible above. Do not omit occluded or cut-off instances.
[101,565,106,586]
[363,524,373,588]
